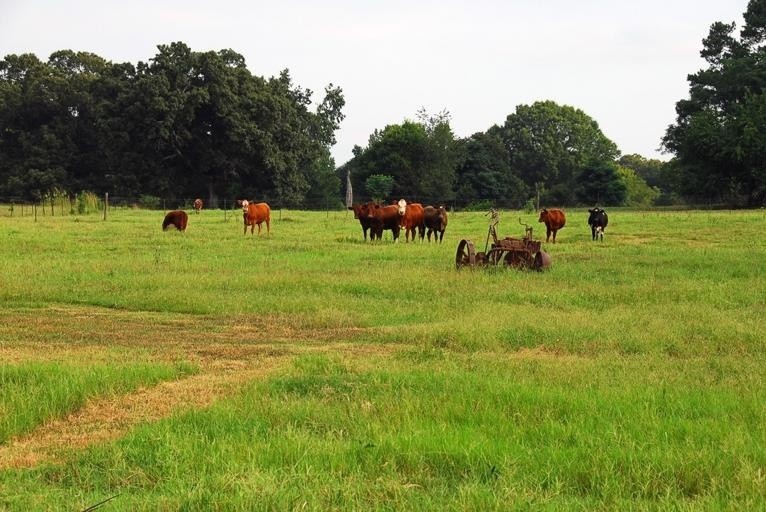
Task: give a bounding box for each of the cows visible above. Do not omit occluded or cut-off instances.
[162,210,189,232]
[424,205,448,243]
[587,207,609,242]
[361,201,400,241]
[393,199,426,243]
[237,199,271,236]
[347,203,383,239]
[193,199,203,214]
[538,207,566,244]
